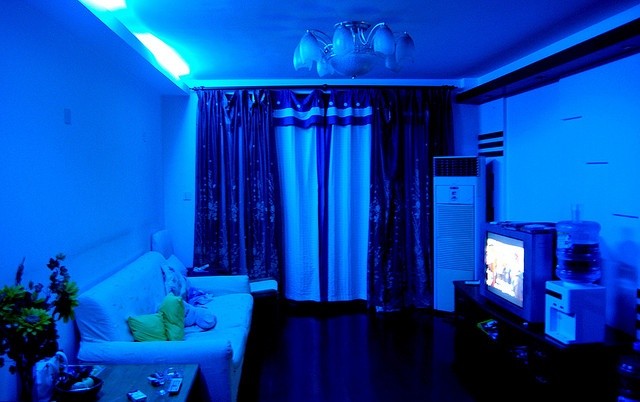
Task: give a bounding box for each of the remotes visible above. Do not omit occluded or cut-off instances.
[465,280,480,285]
[168,377,182,393]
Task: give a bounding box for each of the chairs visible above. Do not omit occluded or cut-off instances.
[153,229,282,330]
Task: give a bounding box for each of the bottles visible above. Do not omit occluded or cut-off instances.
[616,349,640,400]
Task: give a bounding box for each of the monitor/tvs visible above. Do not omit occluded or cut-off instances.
[480,222,556,323]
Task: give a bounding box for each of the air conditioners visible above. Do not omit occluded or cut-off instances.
[434,156,486,310]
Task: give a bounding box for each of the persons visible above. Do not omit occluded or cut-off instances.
[502,263,512,284]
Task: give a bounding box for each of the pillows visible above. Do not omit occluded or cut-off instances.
[157,293,185,340]
[127,313,166,342]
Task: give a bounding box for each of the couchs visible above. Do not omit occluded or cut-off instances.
[76,251,254,402]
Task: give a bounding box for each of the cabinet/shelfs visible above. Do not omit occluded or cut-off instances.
[450,280,572,384]
[75,363,197,402]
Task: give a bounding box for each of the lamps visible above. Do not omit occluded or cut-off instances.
[292,19,416,77]
